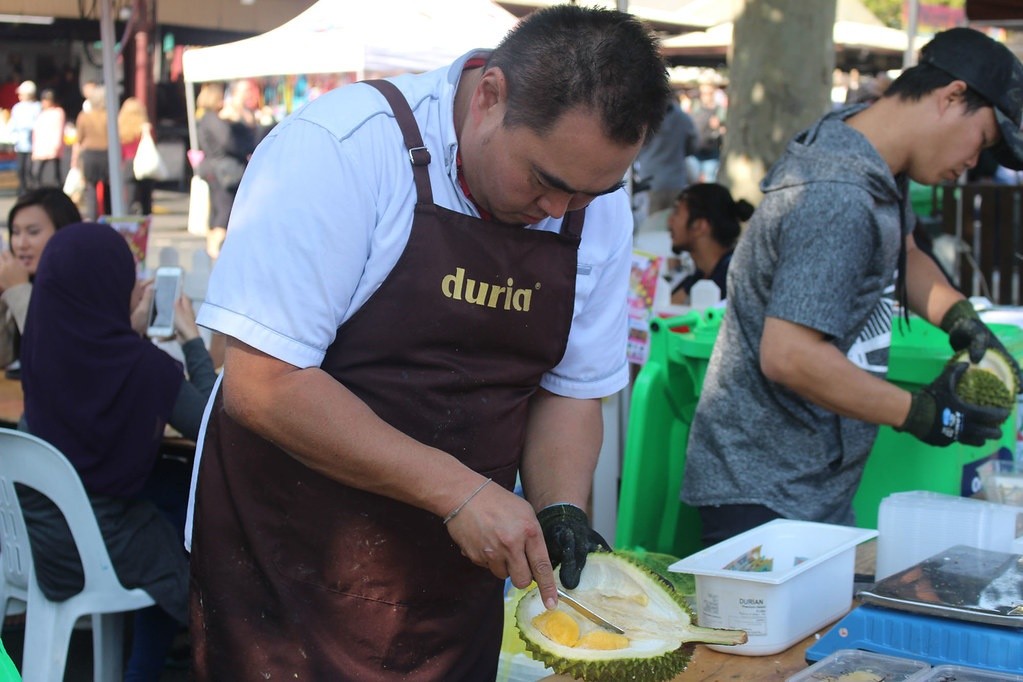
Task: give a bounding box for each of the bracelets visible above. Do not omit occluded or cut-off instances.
[443,478,493,524]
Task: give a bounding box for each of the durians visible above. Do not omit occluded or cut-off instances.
[944,348,1017,417]
[511,548,748,681]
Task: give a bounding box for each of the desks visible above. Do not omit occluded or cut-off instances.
[0,367,198,448]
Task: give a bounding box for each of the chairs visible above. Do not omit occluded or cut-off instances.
[0,428,156,682]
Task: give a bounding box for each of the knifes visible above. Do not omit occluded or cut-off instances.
[532,575,625,636]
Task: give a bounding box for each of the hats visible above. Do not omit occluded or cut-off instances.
[921,27,1023,170]
[18,81,37,94]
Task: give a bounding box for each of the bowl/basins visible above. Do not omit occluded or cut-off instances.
[781,647,1023,682]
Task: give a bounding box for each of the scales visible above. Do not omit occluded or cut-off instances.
[804,543,1023,675]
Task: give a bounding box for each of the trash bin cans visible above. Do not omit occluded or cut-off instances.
[907,178,961,219]
[613,302,1023,594]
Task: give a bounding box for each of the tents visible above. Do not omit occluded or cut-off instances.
[182,0,524,234]
[519,0,931,75]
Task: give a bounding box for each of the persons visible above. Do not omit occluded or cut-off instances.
[0,186,83,372]
[638,60,724,213]
[669,27,1023,558]
[70,78,154,268]
[193,83,246,257]
[6,80,64,196]
[20,221,223,682]
[184,0,669,682]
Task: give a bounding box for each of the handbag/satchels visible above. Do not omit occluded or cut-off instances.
[133,124,164,179]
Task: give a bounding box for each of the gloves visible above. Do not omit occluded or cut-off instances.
[940,300,1023,393]
[893,363,1010,446]
[538,503,614,590]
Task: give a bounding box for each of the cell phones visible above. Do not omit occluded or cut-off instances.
[147,267,183,338]
